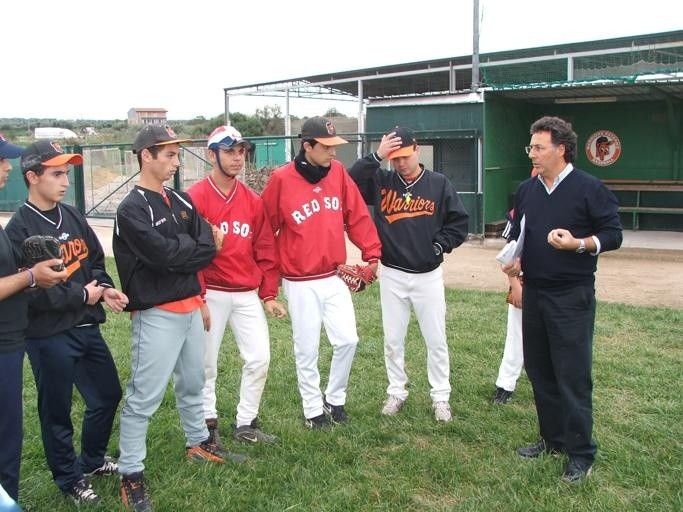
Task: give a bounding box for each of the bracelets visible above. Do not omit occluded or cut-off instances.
[21,267,36,289]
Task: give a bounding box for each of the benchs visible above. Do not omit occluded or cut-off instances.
[598,173,681,231]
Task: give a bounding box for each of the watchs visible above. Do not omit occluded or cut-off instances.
[576,238,585,253]
[432,244,441,257]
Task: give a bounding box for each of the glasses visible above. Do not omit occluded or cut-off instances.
[525,145,552,155]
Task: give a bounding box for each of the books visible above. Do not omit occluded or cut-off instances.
[495,214,525,268]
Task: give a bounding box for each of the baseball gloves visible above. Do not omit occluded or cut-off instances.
[335,265,377,291]
[21,235,64,273]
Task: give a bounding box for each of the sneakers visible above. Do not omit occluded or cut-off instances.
[305,414,331,431]
[64,478,101,507]
[121,473,152,510]
[205,418,221,447]
[493,388,592,484]
[433,400,451,422]
[231,418,278,445]
[382,396,404,416]
[323,396,348,425]
[84,456,120,477]
[185,444,247,465]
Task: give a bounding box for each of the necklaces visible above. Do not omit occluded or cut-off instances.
[402,176,416,205]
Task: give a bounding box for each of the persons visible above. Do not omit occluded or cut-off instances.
[0,131,67,504]
[114,126,244,510]
[497,116,622,481]
[184,125,288,467]
[346,126,468,422]
[491,166,535,405]
[6,138,130,506]
[259,117,382,425]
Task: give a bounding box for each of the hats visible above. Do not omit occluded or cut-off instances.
[298,117,349,146]
[388,126,414,160]
[1,136,24,160]
[207,125,252,151]
[20,139,84,174]
[132,124,193,155]
